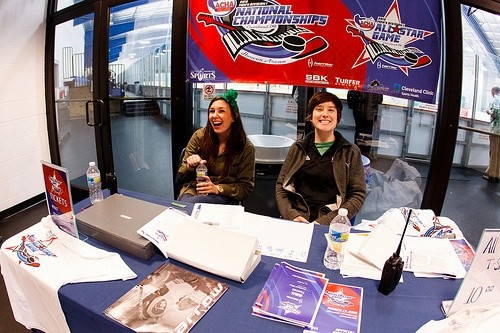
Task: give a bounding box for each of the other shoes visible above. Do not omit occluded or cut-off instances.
[483,175,495,181]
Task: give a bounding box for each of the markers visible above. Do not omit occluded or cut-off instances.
[194,205,201,219]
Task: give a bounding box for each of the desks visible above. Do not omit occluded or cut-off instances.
[0,186,464,333]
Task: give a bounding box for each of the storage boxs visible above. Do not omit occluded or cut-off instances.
[74,193,168,260]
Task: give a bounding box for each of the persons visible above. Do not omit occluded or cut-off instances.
[276,92,366,226]
[482,87,500,178]
[178,89,256,207]
[350,90,383,160]
[292,86,326,140]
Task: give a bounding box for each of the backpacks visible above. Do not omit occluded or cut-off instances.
[347,90,362,109]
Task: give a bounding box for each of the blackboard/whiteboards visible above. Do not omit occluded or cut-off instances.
[445,229,500,318]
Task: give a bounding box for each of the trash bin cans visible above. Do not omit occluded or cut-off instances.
[242,134,295,218]
[350,154,371,226]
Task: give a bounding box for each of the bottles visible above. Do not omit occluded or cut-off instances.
[323,208,352,270]
[86,162,104,205]
[196,160,208,197]
